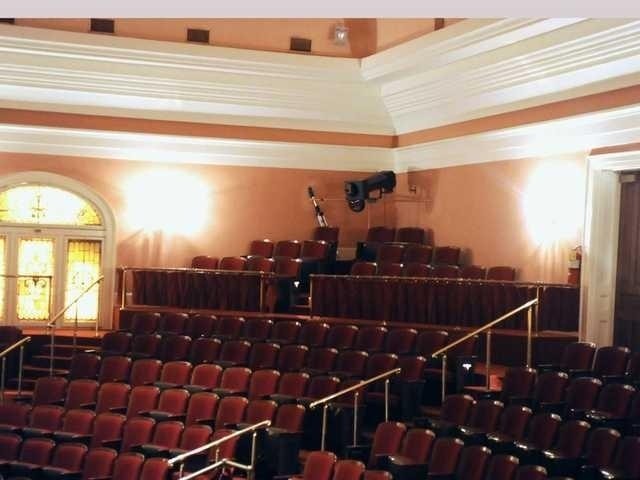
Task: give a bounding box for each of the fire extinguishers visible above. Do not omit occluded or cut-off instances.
[568,246,582,284]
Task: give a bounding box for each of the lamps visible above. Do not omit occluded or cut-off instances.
[332,22,350,48]
